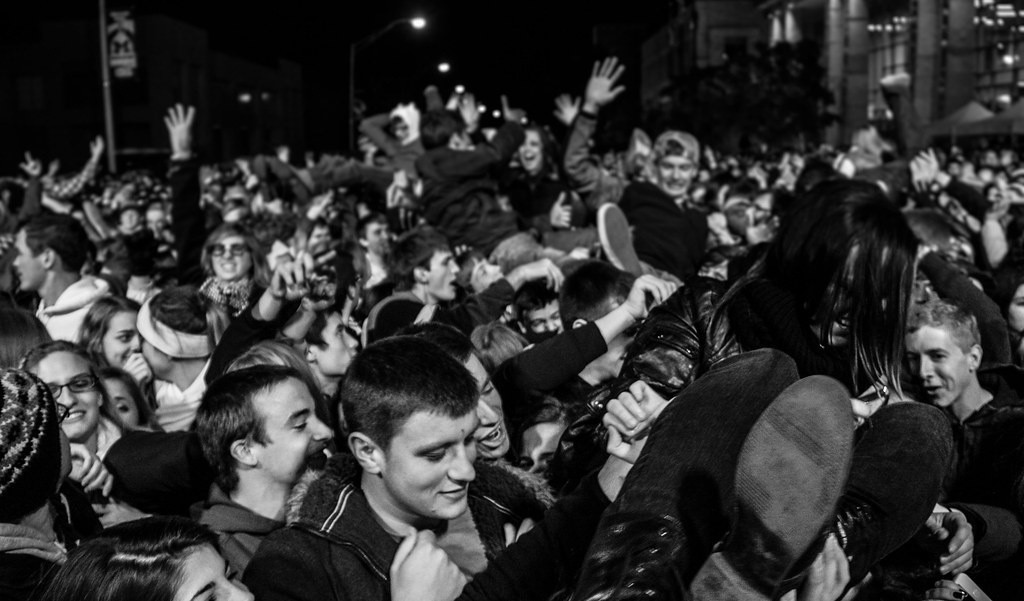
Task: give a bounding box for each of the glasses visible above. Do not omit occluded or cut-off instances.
[205,243,252,256]
[54,400,69,425]
[48,375,100,401]
[660,160,692,170]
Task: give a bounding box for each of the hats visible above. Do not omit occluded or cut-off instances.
[652,130,700,164]
[0,365,61,524]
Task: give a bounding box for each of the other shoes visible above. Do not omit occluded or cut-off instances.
[689,374,855,601]
[567,346,801,601]
[833,402,954,589]
[588,202,643,278]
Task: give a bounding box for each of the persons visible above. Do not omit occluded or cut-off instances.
[0,55,1024,601]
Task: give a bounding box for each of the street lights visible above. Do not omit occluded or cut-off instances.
[347,16,429,156]
